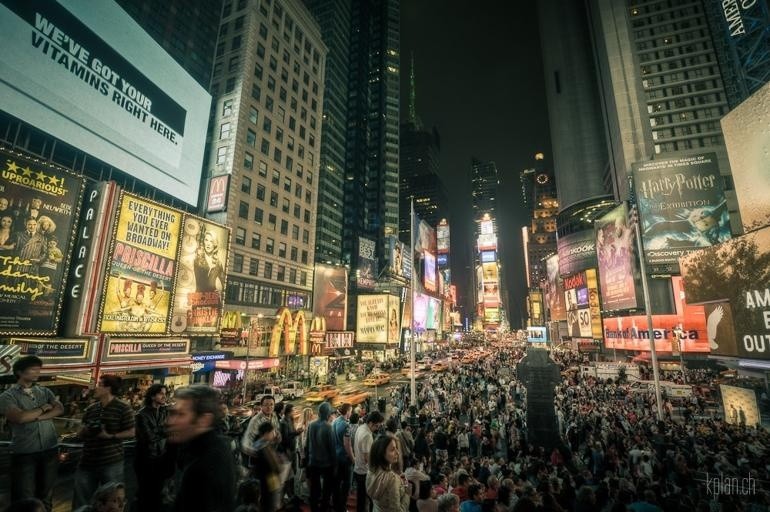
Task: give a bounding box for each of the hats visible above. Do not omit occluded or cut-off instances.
[318,402,337,417]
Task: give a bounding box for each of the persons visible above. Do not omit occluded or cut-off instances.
[111,284,165,332]
[614,215,631,268]
[144,280,164,331]
[0,339,769,511]
[117,274,133,330]
[595,227,615,307]
[191,229,225,326]
[1,194,64,289]
[567,292,576,310]
[392,245,401,268]
[568,312,580,337]
[395,254,403,276]
[391,308,399,339]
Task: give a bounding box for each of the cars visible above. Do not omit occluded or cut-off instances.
[228,342,499,431]
[56,431,81,478]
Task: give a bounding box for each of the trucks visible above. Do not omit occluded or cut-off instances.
[662,383,719,406]
[561,361,639,382]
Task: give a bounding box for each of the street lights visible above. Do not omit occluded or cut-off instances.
[673,326,686,384]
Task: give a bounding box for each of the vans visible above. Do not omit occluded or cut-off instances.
[628,379,675,393]
